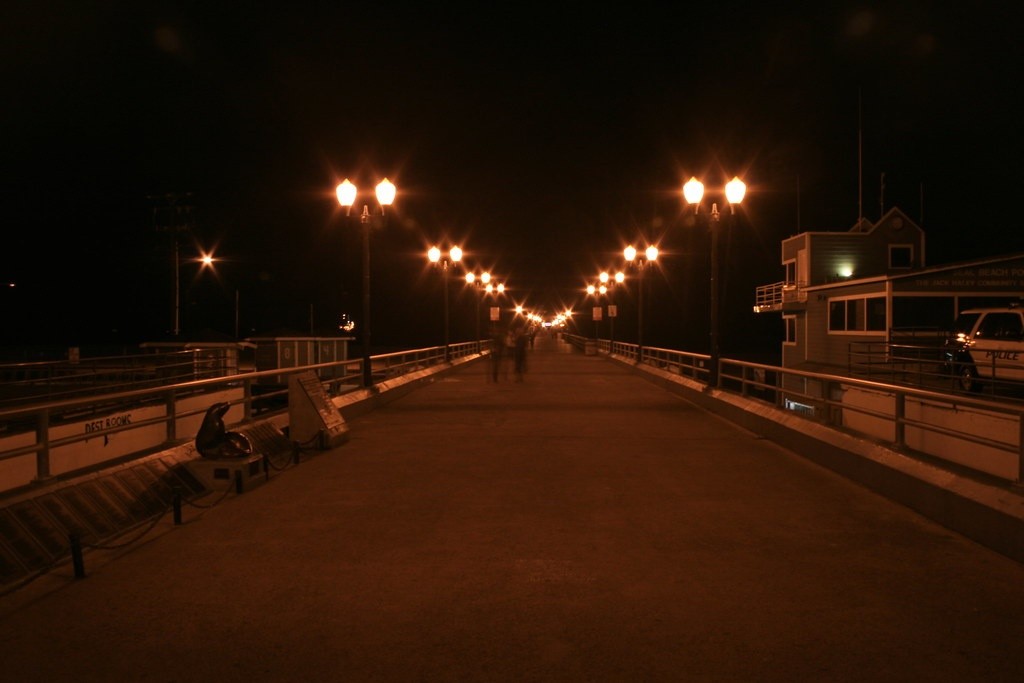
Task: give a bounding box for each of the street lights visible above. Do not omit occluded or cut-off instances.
[683,176,749,387]
[484,261,629,339]
[335,176,401,389]
[621,244,661,364]
[427,243,464,360]
[462,269,492,351]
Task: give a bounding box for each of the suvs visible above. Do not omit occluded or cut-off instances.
[944,300,1023,397]
